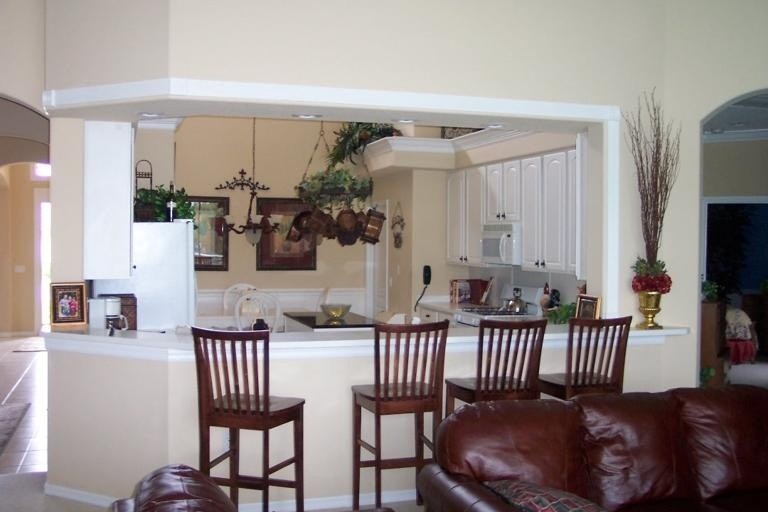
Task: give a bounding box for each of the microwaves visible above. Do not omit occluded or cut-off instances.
[481,222,520,266]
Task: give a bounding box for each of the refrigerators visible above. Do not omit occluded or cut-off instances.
[94,218,197,331]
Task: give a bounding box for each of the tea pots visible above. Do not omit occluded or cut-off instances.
[507,286,524,313]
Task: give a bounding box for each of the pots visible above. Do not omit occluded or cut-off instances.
[284,198,385,247]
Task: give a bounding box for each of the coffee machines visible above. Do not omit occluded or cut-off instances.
[88,297,128,331]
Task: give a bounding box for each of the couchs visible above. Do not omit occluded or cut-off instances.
[407,386,766,512]
[101,464,240,512]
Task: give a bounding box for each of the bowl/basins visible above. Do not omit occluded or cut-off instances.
[319,304,351,320]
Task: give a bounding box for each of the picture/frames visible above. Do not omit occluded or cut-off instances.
[573,295,599,320]
[48,282,86,324]
[182,195,231,270]
[253,194,316,271]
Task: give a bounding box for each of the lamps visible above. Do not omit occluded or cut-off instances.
[212,117,279,246]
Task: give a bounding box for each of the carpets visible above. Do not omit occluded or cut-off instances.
[1,401,30,455]
[13,332,45,352]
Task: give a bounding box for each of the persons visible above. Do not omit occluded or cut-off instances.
[59,295,77,316]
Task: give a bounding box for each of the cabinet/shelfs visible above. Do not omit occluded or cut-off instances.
[446,168,506,270]
[562,147,577,274]
[483,159,517,222]
[418,305,440,325]
[702,304,729,371]
[518,152,562,272]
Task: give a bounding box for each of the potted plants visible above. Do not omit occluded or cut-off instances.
[621,89,683,329]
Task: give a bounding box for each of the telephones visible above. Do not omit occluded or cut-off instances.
[424,266,430,284]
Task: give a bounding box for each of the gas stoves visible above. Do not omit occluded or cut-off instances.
[453,305,534,327]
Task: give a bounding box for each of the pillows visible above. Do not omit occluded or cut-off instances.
[476,466,606,511]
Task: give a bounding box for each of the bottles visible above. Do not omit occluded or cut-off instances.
[166,184,176,222]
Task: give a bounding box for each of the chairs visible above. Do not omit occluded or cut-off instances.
[724,292,757,363]
[222,281,260,314]
[314,286,331,312]
[191,324,312,511]
[446,312,549,411]
[233,291,283,330]
[539,314,631,399]
[351,316,447,512]
